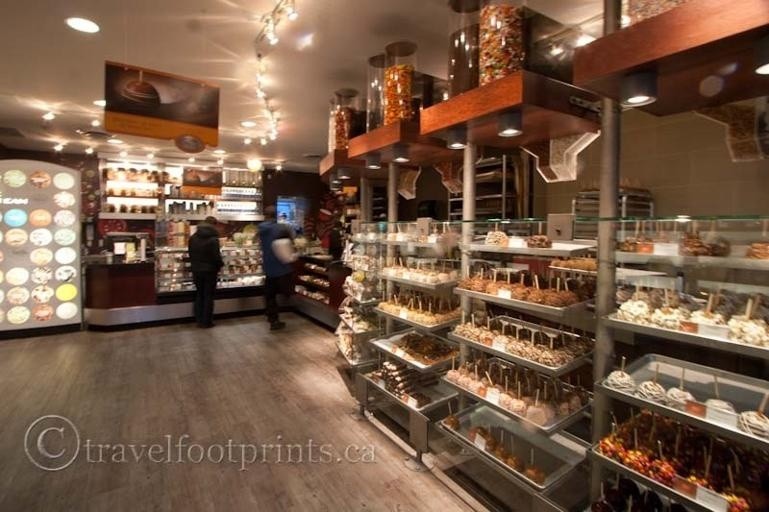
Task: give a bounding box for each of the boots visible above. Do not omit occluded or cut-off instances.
[271,319,286,330]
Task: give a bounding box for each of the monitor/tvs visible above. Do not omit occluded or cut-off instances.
[106,235,138,252]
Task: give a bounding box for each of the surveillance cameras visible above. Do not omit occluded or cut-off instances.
[256,42,281,59]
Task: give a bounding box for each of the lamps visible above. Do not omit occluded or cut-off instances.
[751,33,769,76]
[619,67,657,109]
[263,0,298,46]
[318,107,525,218]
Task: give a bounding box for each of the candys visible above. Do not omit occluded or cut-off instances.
[479,3,526,87]
[120,70,160,106]
[378,219,769,512]
[382,63,415,125]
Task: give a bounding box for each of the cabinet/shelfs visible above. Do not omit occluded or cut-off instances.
[328,147,768,512]
[71,156,347,338]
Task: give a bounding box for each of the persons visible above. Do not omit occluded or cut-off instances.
[187,215,225,328]
[257,204,305,331]
